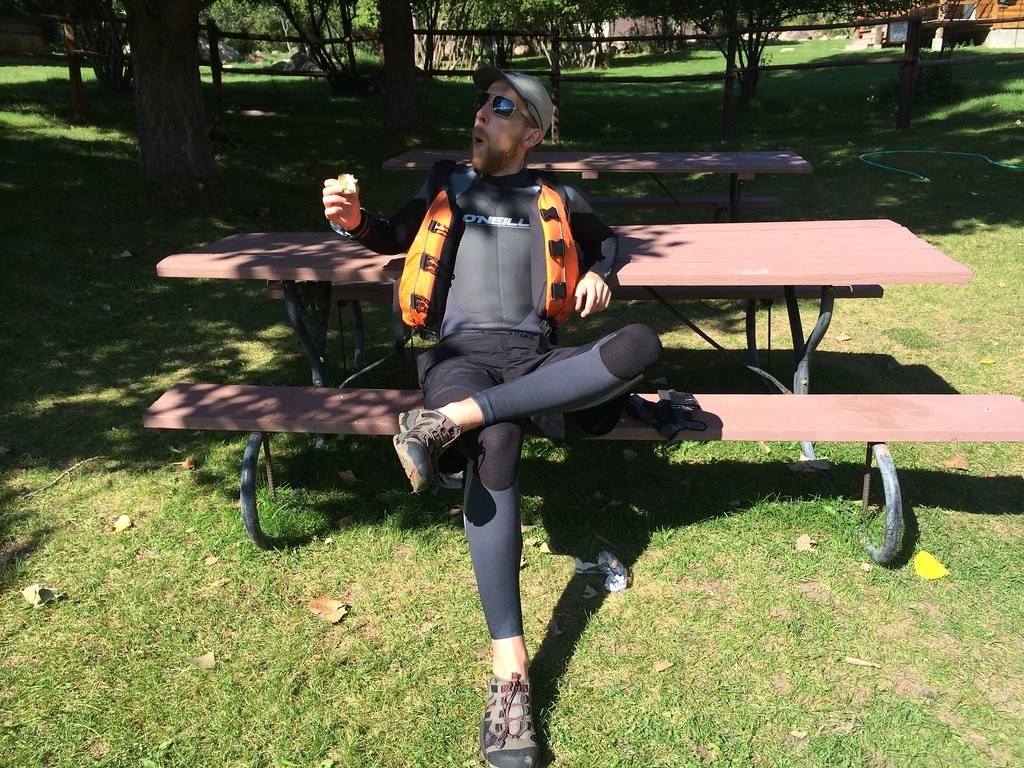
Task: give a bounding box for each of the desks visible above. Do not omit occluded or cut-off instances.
[155,218,972,469]
[381,148,816,217]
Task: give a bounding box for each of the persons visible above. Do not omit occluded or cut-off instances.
[320,64,664,768]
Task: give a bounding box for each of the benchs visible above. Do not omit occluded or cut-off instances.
[138,381,1023,565]
[268,285,883,388]
[585,193,782,225]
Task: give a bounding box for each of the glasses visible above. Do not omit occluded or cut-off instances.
[475,91,534,127]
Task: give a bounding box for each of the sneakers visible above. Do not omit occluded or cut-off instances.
[393,405,462,495]
[479,675,540,768]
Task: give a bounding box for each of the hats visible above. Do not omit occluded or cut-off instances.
[473,66,553,137]
